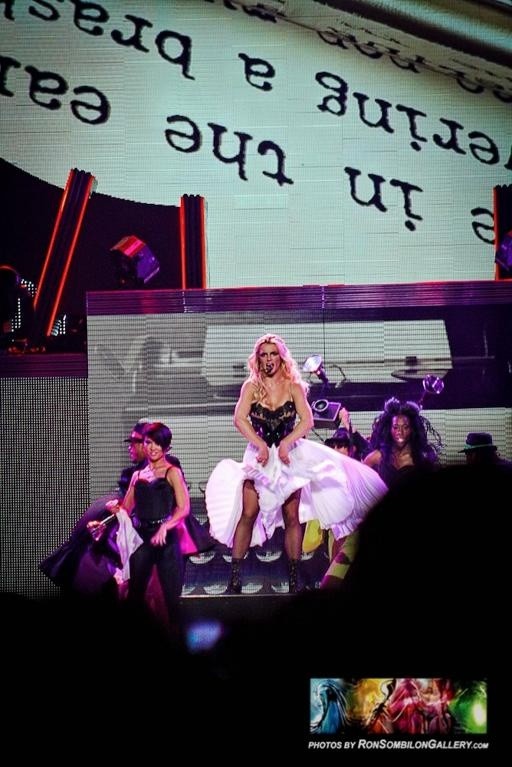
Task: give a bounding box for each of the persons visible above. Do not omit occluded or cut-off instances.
[324,427,362,464]
[362,397,440,489]
[457,433,512,466]
[112,421,192,629]
[204,334,390,593]
[83,422,198,605]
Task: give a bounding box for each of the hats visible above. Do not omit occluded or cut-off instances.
[124,417,152,443]
[457,432,497,451]
[325,427,351,444]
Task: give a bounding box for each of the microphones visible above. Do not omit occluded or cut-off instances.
[264,366,272,373]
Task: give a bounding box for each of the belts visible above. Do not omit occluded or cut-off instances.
[144,518,166,524]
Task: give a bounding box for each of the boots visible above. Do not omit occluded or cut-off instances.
[290,556,300,595]
[225,559,244,596]
